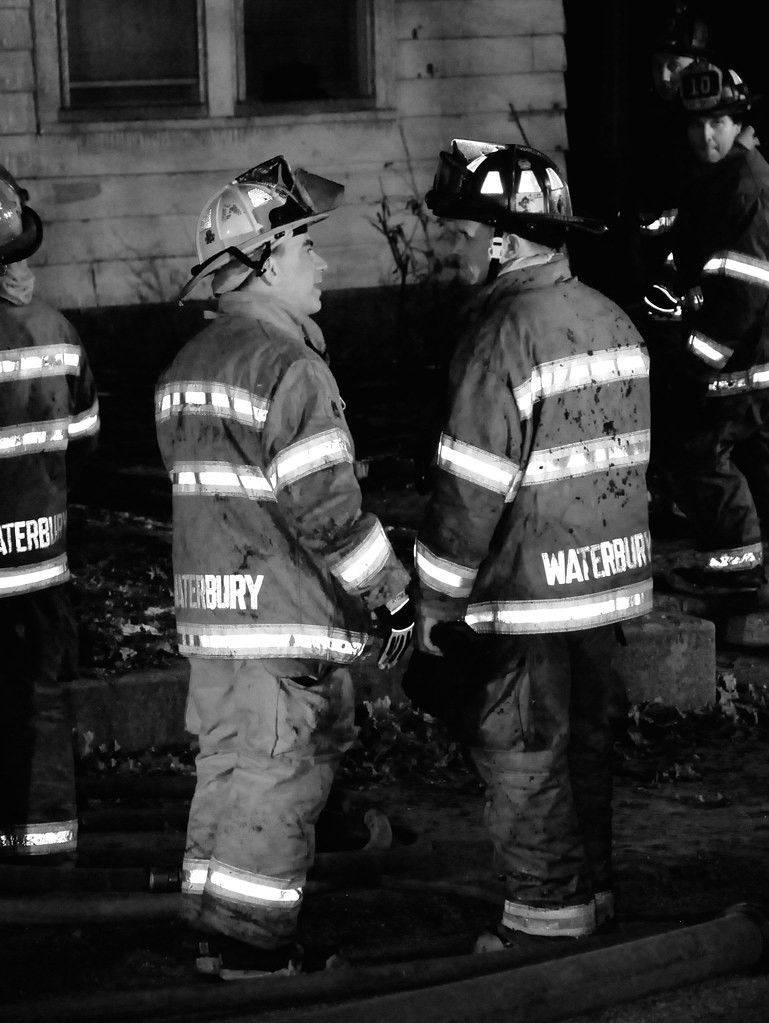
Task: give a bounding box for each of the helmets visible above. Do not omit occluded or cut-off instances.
[0,162,43,265]
[177,155,328,301]
[423,138,608,250]
[655,53,753,124]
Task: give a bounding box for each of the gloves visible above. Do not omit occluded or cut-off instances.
[376,599,416,670]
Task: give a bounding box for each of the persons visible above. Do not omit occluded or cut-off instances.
[152,157,414,976]
[623,57,769,588]
[411,140,655,952]
[0,166,103,870]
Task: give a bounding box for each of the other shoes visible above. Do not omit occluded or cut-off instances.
[470,916,570,956]
[671,559,765,590]
[192,927,341,978]
